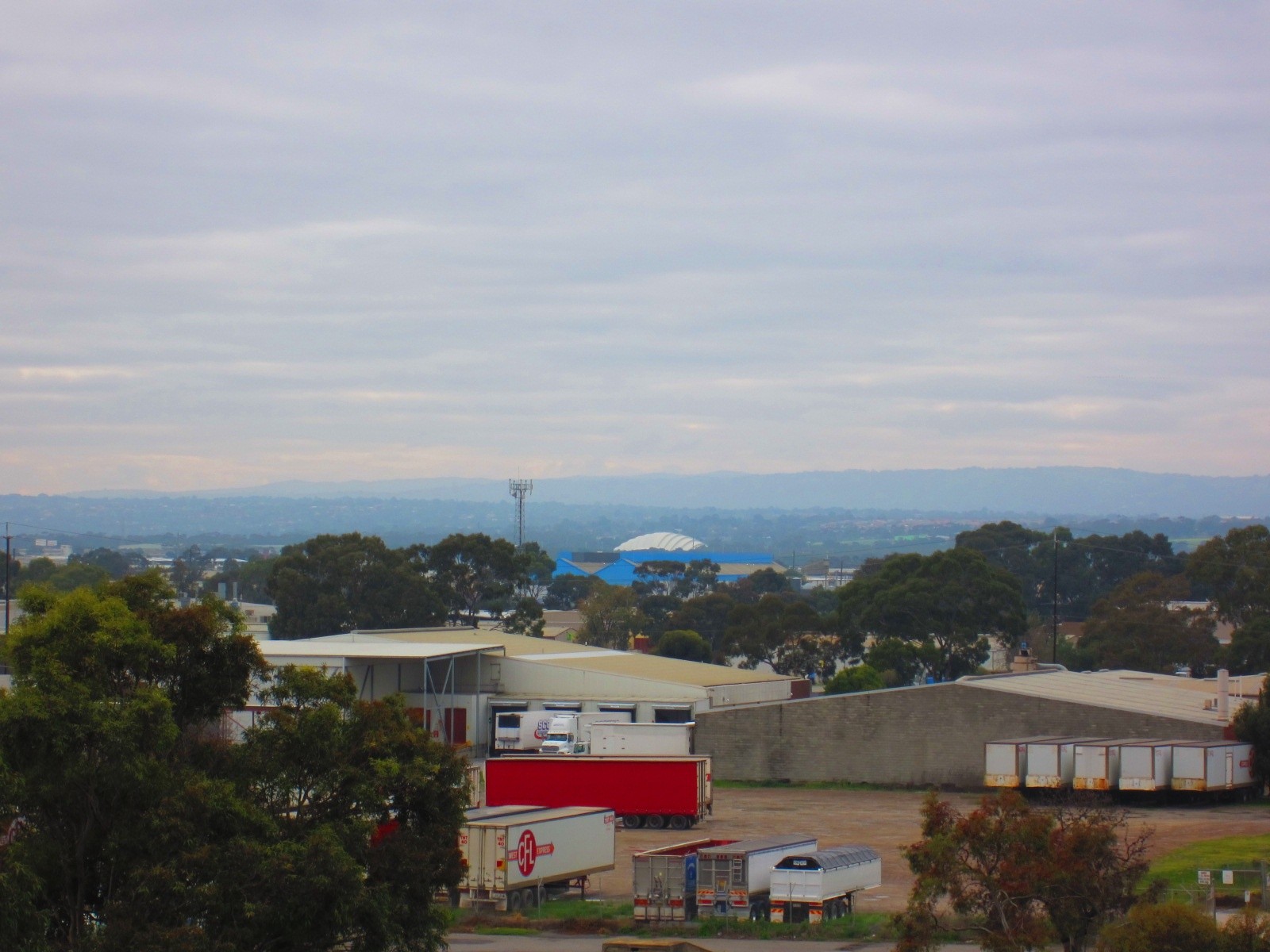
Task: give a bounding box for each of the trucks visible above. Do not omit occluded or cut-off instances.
[539,711,632,756]
